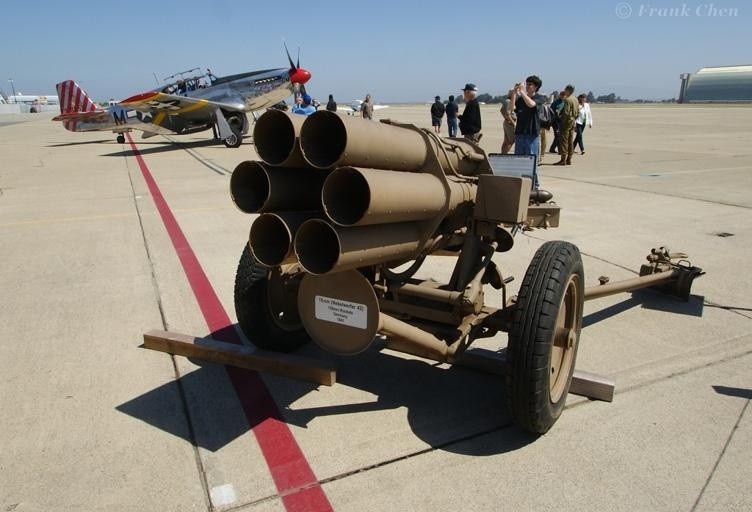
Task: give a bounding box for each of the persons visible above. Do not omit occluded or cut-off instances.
[291,94,321,115]
[326,94,337,111]
[500,75,592,189]
[455,83,482,145]
[361,95,374,119]
[446,95,458,137]
[430,96,445,133]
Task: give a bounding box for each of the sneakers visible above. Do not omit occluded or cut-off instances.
[539,149,587,166]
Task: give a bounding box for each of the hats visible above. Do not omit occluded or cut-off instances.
[461,84,478,91]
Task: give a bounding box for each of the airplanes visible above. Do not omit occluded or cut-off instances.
[336,100,389,117]
[51,41,311,148]
